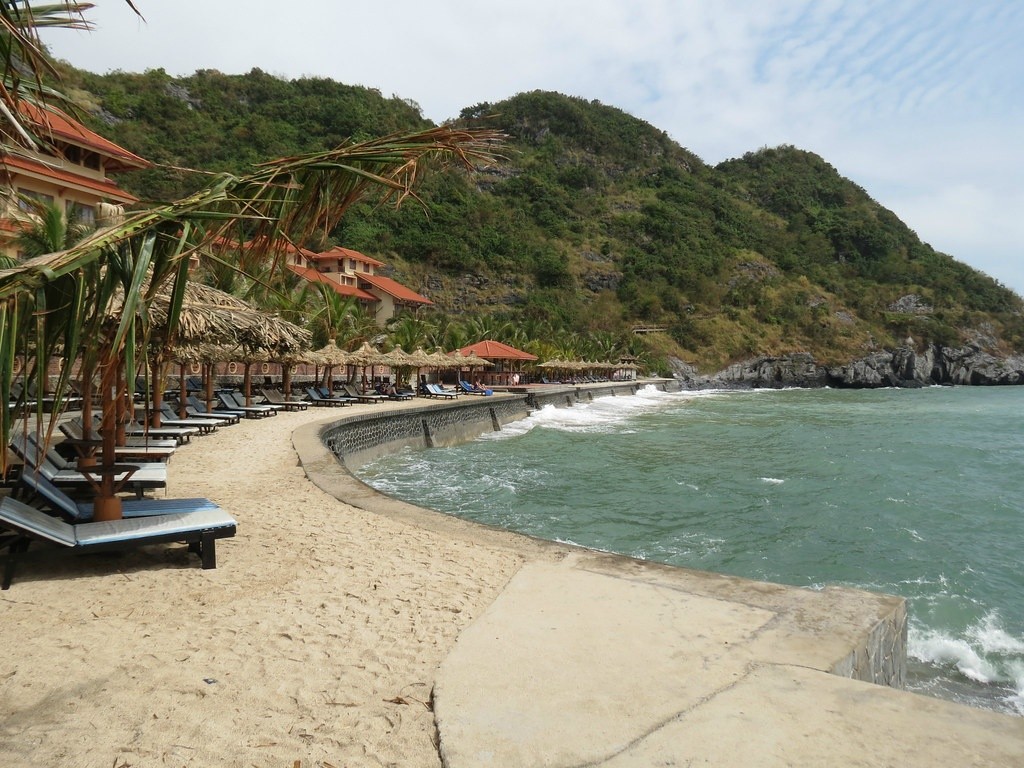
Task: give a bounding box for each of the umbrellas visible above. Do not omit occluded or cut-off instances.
[263,339,495,411]
[536,356,641,383]
[0,202,313,561]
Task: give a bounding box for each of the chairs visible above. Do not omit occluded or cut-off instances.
[542,376,632,384]
[0,376,492,590]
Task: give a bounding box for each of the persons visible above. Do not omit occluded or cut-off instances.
[475,379,487,391]
[380,383,399,395]
